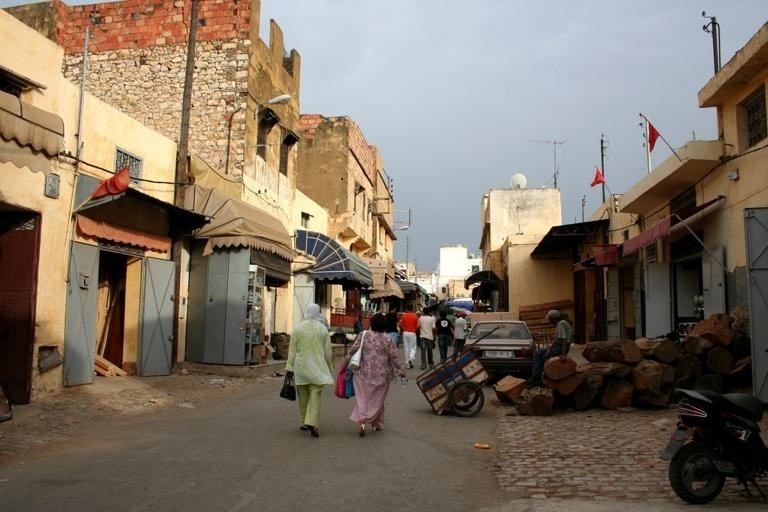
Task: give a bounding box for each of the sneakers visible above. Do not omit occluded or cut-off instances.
[409,360,415,369]
[306,424,320,438]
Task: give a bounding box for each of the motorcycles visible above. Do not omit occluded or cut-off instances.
[658,385,767,506]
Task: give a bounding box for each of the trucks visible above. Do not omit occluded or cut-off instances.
[328,305,373,345]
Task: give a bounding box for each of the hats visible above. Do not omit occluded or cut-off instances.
[544,310,560,319]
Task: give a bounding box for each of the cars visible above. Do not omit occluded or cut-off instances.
[462,320,538,374]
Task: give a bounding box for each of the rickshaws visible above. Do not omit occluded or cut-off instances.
[415,322,502,416]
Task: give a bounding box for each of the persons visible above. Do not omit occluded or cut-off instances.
[385,304,468,369]
[335,313,406,438]
[527,309,574,389]
[280,303,335,438]
[354,315,362,335]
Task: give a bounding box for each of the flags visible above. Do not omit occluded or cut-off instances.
[92,165,130,200]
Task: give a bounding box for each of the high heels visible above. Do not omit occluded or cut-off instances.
[359,424,367,437]
[372,423,383,431]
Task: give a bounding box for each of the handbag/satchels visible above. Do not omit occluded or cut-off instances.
[347,348,361,370]
[280,384,297,401]
[336,369,356,400]
